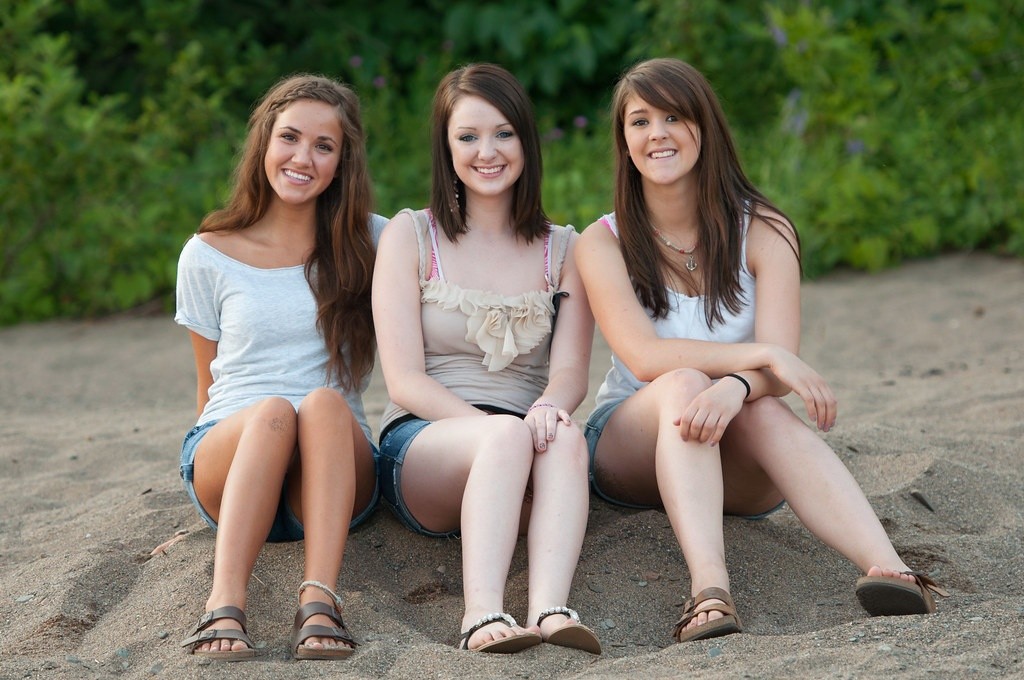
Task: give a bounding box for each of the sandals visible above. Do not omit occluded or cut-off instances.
[537,606,602,655]
[855,571,951,617]
[460,612,542,651]
[291,601,362,660]
[670,587,743,642]
[181,605,255,662]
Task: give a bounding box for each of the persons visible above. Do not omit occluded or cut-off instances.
[574,57,950,642]
[371,63,602,655]
[174,74,392,661]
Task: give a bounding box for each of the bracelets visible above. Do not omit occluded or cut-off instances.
[726,373,750,398]
[530,403,554,410]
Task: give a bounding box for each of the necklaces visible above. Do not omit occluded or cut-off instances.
[649,223,699,272]
